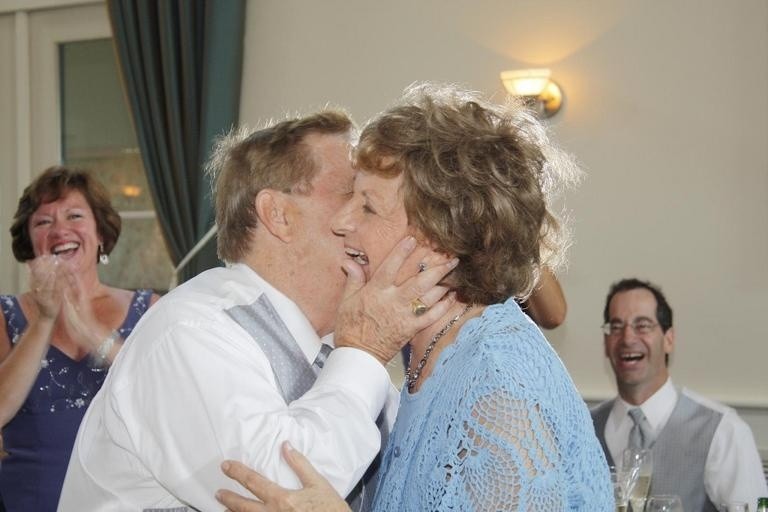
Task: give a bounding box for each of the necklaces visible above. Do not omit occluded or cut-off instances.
[405,299,477,388]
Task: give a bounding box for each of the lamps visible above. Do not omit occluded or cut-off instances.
[500,64,563,124]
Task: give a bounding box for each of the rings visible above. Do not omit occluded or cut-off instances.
[411,297,428,318]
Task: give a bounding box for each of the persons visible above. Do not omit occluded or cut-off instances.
[1,162,163,511]
[211,77,621,510]
[54,102,460,511]
[518,261,567,329]
[588,278,768,512]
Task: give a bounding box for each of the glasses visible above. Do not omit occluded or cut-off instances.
[601,319,660,335]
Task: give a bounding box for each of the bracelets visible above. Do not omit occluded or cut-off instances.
[97,327,121,359]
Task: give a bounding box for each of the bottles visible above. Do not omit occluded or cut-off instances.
[756,496,768,512]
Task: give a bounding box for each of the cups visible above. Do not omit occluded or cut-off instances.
[611,445,683,511]
[719,501,748,512]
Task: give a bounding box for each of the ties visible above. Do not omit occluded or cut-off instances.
[627,407,655,476]
